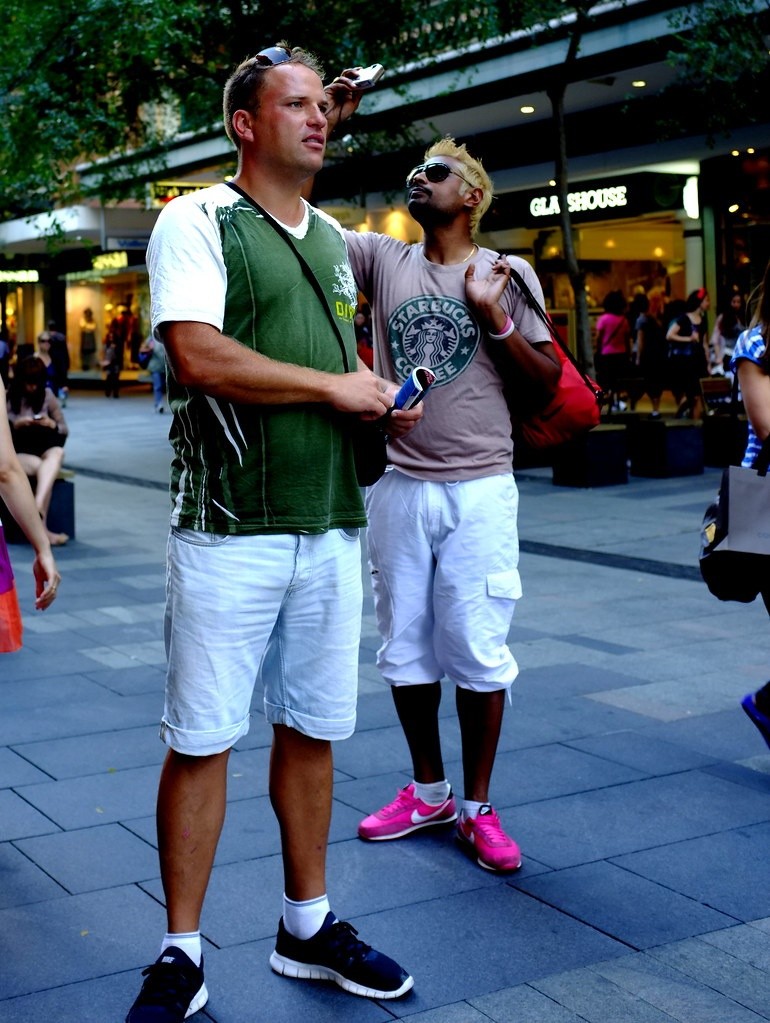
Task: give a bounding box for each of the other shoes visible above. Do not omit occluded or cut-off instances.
[155,406,164,414]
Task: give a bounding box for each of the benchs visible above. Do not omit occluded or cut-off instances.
[698,378,748,463]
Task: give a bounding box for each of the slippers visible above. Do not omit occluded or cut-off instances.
[740,691,770,749]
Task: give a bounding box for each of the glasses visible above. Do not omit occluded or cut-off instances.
[404,162,475,189]
[242,46,293,68]
[40,339,54,343]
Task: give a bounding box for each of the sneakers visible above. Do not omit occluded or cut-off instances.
[124,947,211,1023]
[454,804,523,873]
[357,784,459,842]
[268,910,415,1000]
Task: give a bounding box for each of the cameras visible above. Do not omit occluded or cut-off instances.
[347,64,385,91]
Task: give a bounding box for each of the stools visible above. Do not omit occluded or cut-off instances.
[1,467,77,545]
[550,424,631,489]
[640,416,702,479]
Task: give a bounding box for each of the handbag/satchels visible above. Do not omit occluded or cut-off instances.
[593,350,608,374]
[349,414,395,485]
[699,433,769,604]
[488,263,605,453]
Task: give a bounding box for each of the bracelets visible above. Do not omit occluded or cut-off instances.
[54,421,59,434]
[487,314,516,340]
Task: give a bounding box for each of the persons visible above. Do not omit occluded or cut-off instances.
[0,320,73,654]
[79,305,171,415]
[591,286,749,419]
[730,266,770,743]
[301,68,563,872]
[123,44,416,1023]
